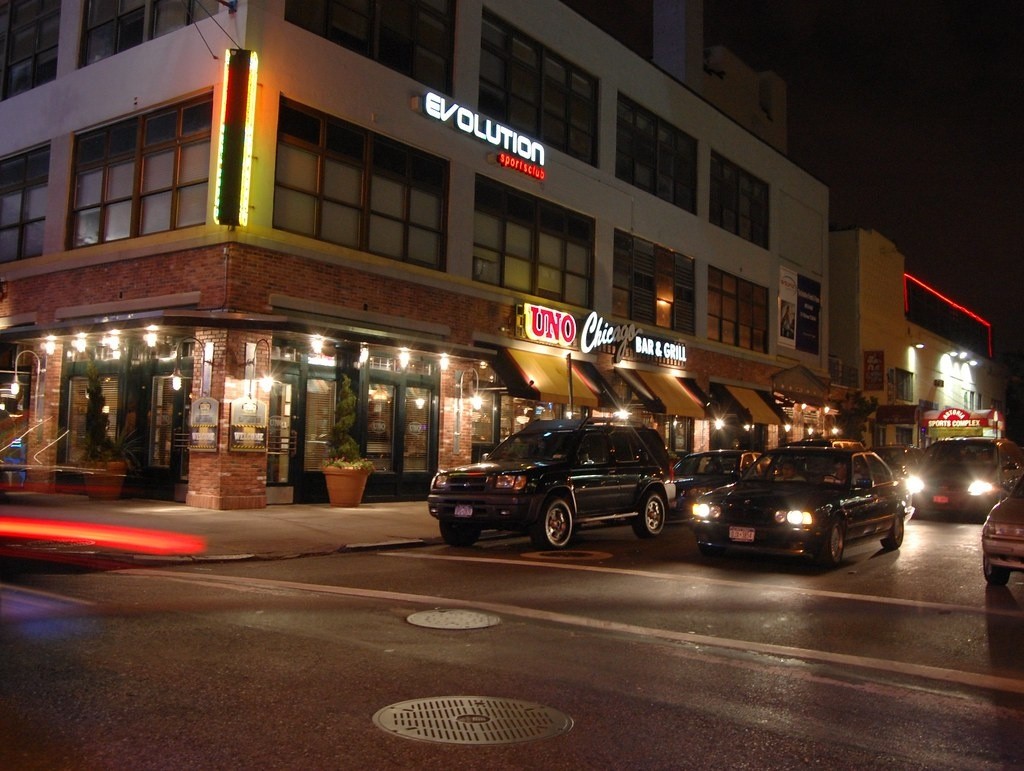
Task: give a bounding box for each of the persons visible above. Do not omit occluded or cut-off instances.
[829,461,857,485]
[774,461,806,481]
[782,305,793,336]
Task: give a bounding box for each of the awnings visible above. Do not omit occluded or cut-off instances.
[714,384,792,425]
[614,367,715,419]
[494,348,623,413]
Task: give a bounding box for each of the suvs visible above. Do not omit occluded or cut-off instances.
[430,418,675,549]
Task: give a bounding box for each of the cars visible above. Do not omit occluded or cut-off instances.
[869,437,1022,521]
[982,471,1024,587]
[670,440,905,572]
